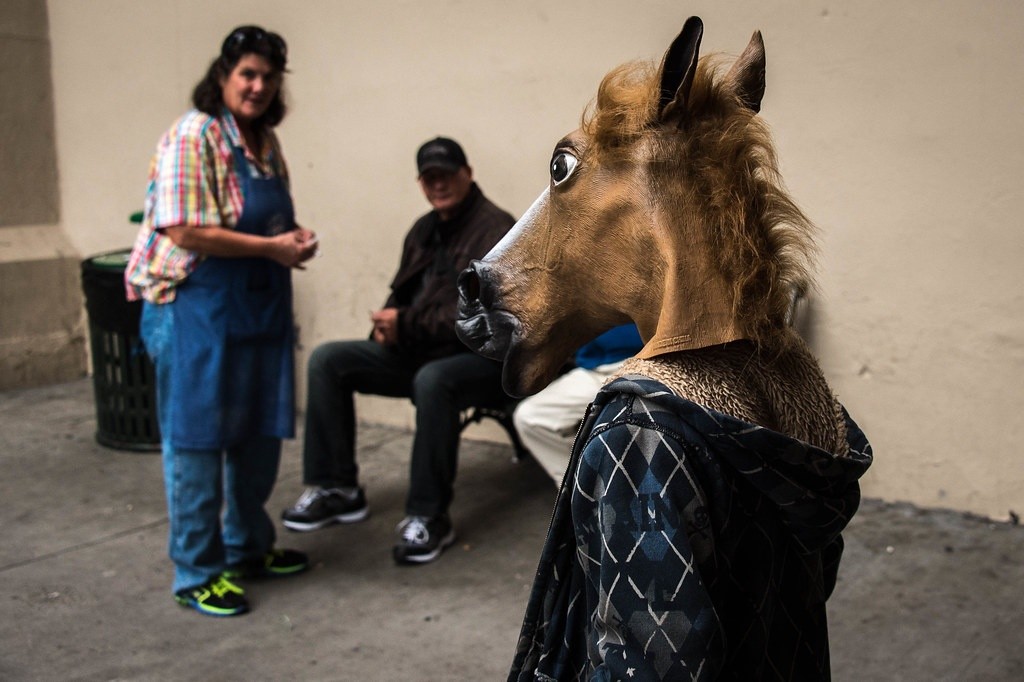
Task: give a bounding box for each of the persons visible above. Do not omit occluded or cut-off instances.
[124,25,319,616]
[508,324,873,682]
[513,323,644,493]
[280,137,518,566]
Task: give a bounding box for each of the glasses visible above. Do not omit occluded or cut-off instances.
[229,30,289,56]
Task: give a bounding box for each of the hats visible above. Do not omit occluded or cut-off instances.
[417,137,469,181]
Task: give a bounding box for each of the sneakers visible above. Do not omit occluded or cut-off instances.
[174,574,248,617]
[222,547,309,581]
[392,512,456,563]
[280,483,369,530]
